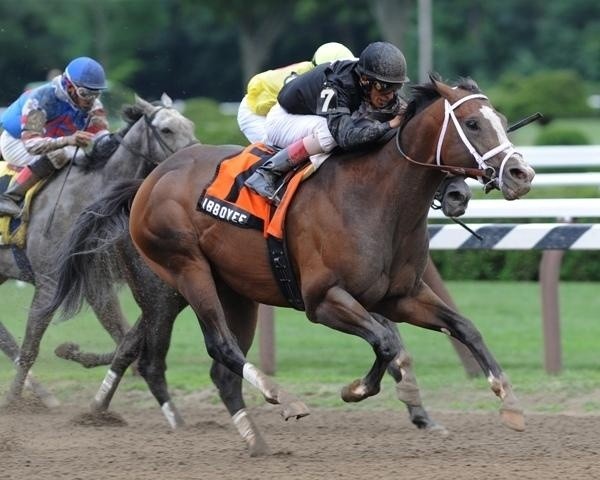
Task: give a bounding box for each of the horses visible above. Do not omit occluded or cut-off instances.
[22,71,536,459]
[53,174,472,439]
[0,91,201,410]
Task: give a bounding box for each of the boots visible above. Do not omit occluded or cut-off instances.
[0,165,44,215]
[243,138,311,207]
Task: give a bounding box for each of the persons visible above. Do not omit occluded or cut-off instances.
[0,56,112,216]
[244,40,403,207]
[238,42,360,145]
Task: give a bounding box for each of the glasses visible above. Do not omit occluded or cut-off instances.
[374,81,404,96]
[76,88,101,103]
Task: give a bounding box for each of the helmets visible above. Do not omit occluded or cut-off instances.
[358,41,409,84]
[311,42,360,67]
[65,56,108,89]
